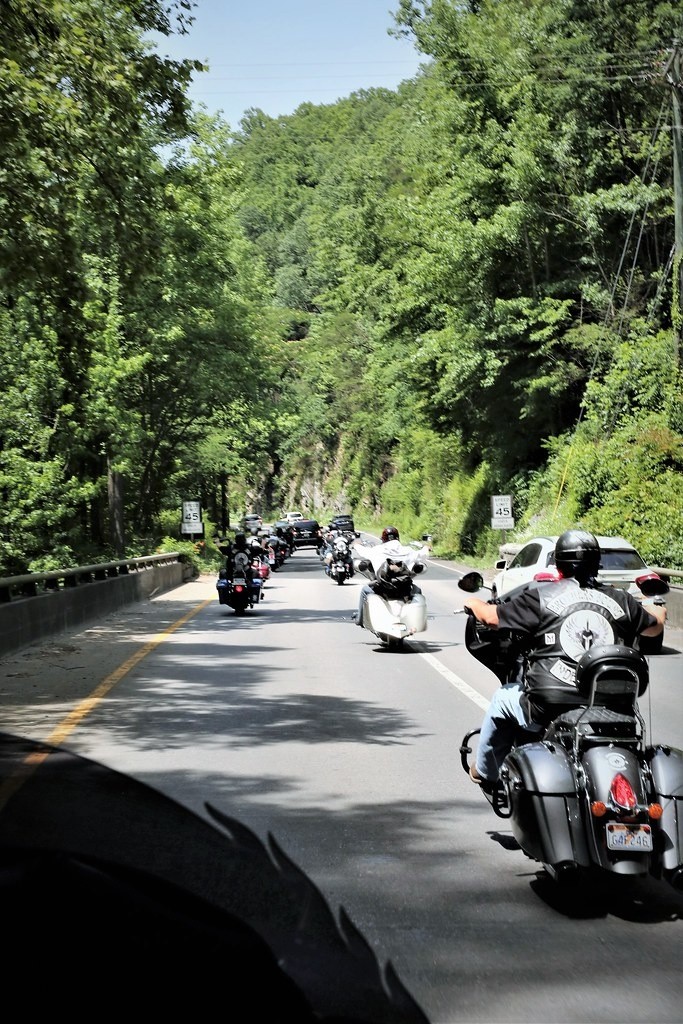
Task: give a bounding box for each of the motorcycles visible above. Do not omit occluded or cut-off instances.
[454,572,683,898]
[320,527,360,585]
[352,545,433,652]
[213,529,297,612]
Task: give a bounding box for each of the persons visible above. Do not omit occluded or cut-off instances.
[463,529,668,783]
[212,526,290,581]
[352,527,433,625]
[317,523,352,574]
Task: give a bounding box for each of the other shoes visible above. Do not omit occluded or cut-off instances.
[469,764,482,783]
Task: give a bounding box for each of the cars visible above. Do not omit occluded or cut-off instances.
[278,512,324,550]
[491,536,666,652]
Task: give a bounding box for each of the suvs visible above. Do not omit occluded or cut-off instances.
[329,515,354,533]
[238,514,262,531]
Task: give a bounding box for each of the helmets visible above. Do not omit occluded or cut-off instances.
[235,532,246,544]
[555,530,603,577]
[337,531,343,536]
[251,527,258,535]
[382,527,399,543]
[269,529,277,535]
